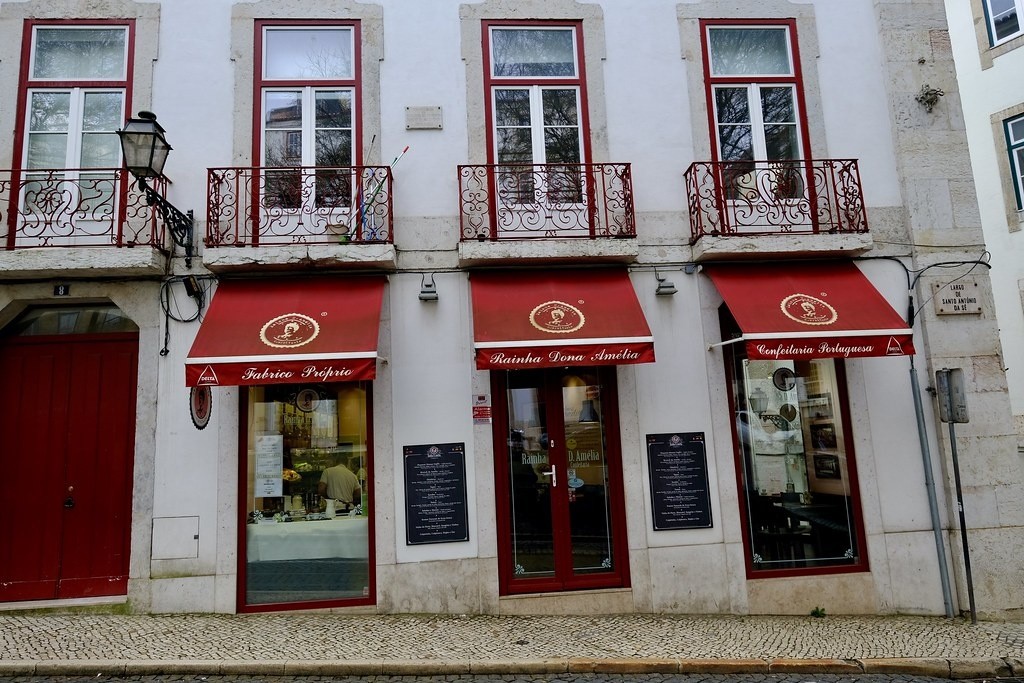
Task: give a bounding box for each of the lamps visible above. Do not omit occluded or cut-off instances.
[418,283,439,301]
[578,386,600,424]
[748,386,785,432]
[654,282,678,295]
[115,111,195,268]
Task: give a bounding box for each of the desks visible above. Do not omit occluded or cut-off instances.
[763,499,856,565]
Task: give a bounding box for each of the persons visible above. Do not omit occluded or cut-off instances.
[351,457,366,492]
[318,457,361,515]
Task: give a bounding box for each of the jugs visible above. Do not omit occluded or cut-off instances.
[325,498,339,517]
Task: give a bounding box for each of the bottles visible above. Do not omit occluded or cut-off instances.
[786,479,794,493]
[761,489,767,496]
[293,495,302,509]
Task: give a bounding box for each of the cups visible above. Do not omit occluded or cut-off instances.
[311,514,322,519]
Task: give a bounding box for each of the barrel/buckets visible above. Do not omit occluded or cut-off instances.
[325,224,350,241]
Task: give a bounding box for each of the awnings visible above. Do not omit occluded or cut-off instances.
[707,265,917,360]
[471,269,655,371]
[185,275,389,387]
